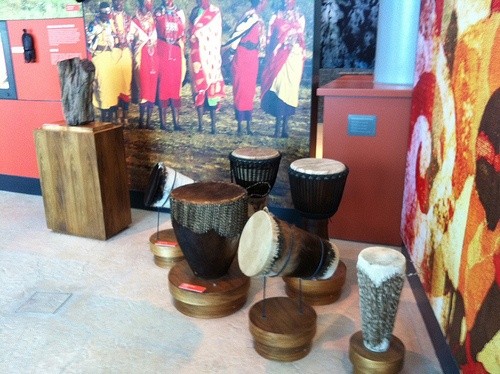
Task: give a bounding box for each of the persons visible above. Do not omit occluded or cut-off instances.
[128,0,159,129]
[222,0,269,135]
[87,2,124,129]
[261,0,306,138]
[108,0,137,124]
[153,0,187,131]
[185,0,224,134]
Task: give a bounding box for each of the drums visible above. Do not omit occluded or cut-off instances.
[167,181,248,280]
[354,245,408,354]
[288,156,350,241]
[236,207,341,282]
[228,146,283,213]
